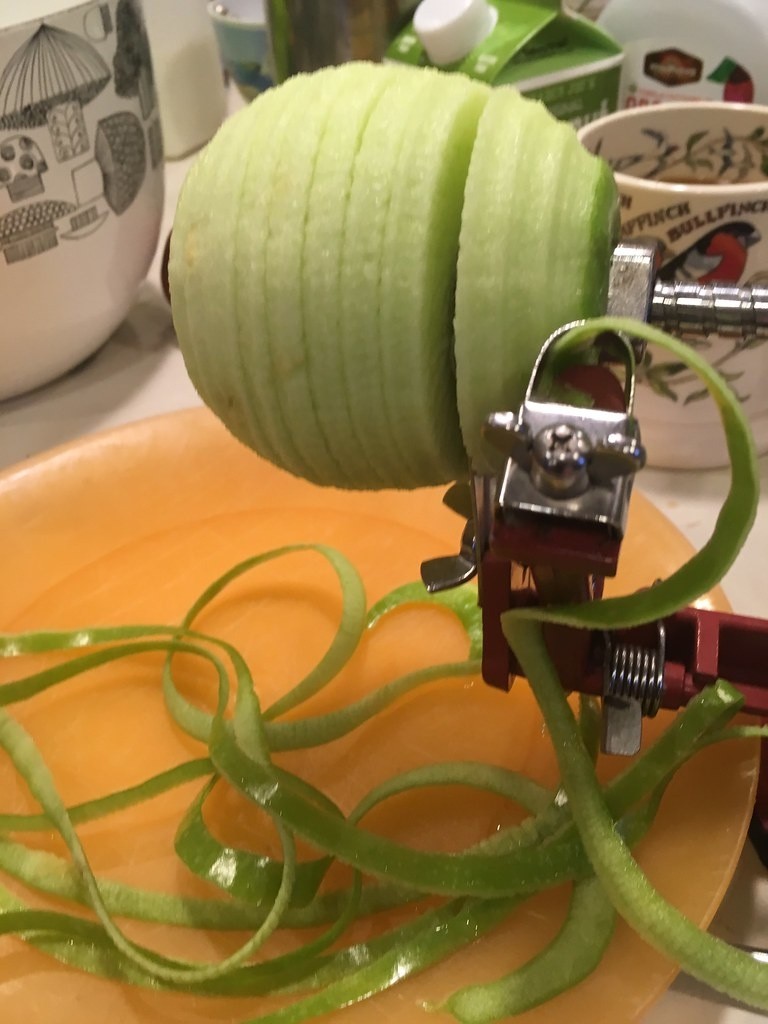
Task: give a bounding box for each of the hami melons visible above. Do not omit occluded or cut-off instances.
[168,61,620,489]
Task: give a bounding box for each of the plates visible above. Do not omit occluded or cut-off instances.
[1,404,763,1023]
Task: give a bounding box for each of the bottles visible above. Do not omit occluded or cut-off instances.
[593,0,767,127]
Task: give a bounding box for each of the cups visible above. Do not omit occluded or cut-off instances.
[140,0,230,161]
[1,1,162,402]
[210,1,278,105]
[577,101,767,472]
